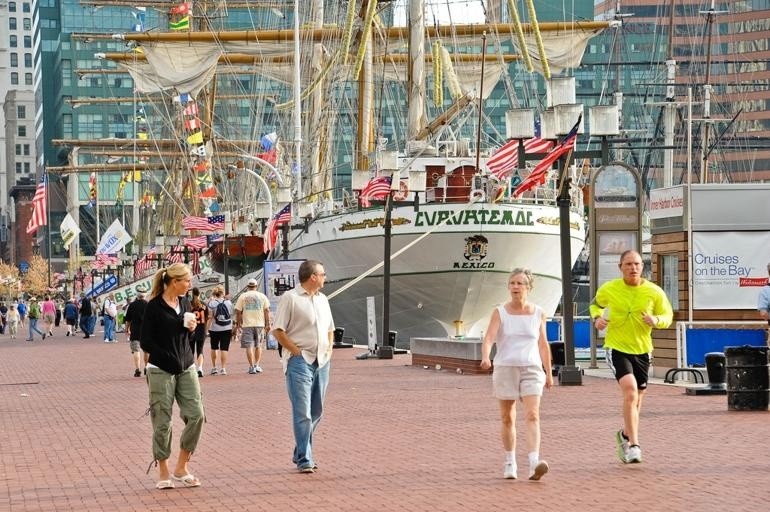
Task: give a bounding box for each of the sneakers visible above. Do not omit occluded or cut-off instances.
[26,330,131,343]
[504,459,519,480]
[616,430,643,463]
[529,460,549,481]
[134,364,263,378]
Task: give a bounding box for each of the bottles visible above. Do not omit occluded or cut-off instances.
[455,336,465,340]
[479,331,483,340]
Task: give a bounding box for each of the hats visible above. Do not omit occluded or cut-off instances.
[247,278,257,287]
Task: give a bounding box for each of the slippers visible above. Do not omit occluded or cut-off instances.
[171,473,201,488]
[155,480,175,490]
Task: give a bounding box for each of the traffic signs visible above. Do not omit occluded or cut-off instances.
[56,185,313,298]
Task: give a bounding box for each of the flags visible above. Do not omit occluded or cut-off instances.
[88,170,97,209]
[166,240,185,263]
[512,113,583,199]
[26,168,49,237]
[135,247,158,276]
[259,151,281,168]
[126,91,160,210]
[132,9,146,21]
[75,267,82,287]
[193,252,201,274]
[89,260,108,271]
[183,234,225,250]
[115,169,126,208]
[263,203,290,256]
[486,118,556,181]
[258,132,279,149]
[174,92,229,214]
[169,2,189,15]
[130,22,144,32]
[179,215,225,230]
[169,16,189,32]
[97,252,118,262]
[357,175,397,210]
[82,270,93,287]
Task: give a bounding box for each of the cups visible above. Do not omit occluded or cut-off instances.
[436,364,442,370]
[455,368,463,374]
[183,311,196,329]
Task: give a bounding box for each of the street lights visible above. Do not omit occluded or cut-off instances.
[234,152,283,183]
[225,163,275,220]
[503,76,620,386]
[348,148,431,360]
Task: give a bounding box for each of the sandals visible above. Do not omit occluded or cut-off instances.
[292,457,318,473]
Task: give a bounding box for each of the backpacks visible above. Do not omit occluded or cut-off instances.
[215,303,233,326]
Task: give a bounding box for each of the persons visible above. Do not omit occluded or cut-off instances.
[1,278,271,378]
[589,251,676,466]
[757,263,770,328]
[273,260,340,472]
[142,263,206,491]
[481,270,553,482]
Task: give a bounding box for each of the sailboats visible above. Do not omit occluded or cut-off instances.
[42,1,621,354]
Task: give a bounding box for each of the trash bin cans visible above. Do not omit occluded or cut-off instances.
[723,343,770,411]
